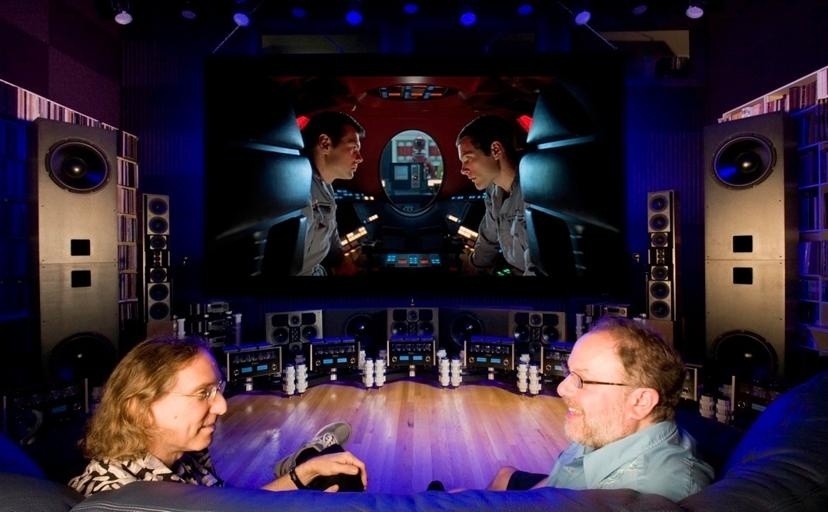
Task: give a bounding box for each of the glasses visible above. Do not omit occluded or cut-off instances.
[562,362,631,390]
[165,379,226,402]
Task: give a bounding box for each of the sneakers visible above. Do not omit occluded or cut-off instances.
[272,421,352,478]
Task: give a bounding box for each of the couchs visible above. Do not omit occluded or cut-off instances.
[0,373,828,512]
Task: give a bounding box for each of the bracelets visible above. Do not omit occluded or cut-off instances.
[287,466,308,492]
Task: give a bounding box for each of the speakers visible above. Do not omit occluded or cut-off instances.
[138,189,682,396]
[27,117,120,388]
[703,110,799,386]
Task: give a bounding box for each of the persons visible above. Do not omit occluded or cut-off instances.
[68,321,374,498]
[427,311,719,505]
[457,113,537,276]
[296,111,367,276]
